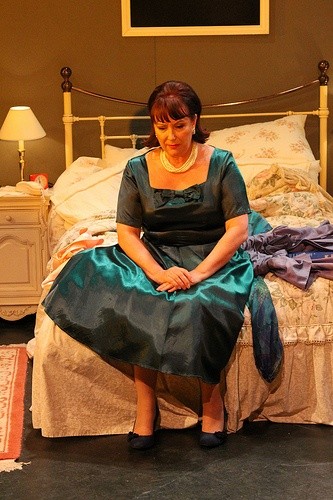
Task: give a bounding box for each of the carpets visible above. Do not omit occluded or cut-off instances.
[0,344,31,472]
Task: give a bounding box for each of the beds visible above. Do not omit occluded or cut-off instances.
[32,60,333,437]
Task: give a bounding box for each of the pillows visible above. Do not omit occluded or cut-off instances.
[103,143,139,169]
[206,115,318,165]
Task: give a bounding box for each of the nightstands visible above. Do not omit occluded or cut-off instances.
[0,185,50,321]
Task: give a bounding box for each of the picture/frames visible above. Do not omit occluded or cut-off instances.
[121,0,269,37]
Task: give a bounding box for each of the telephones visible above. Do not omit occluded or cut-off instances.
[16,182,44,195]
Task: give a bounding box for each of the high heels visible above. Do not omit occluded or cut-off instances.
[200,405,229,448]
[127,399,162,452]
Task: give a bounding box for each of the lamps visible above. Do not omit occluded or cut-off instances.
[0,106,46,181]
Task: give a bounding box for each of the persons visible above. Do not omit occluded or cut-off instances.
[42,81,255,455]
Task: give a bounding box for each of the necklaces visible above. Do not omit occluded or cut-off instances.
[159,141,199,173]
